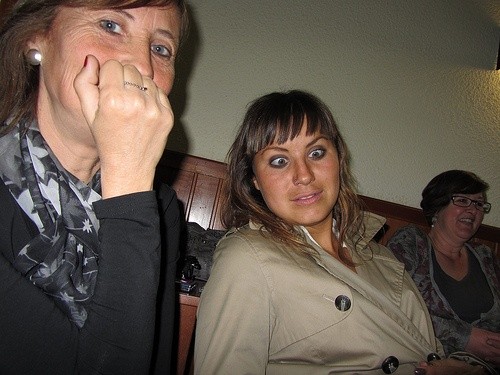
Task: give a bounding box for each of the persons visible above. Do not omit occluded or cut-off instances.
[386,170,500,359]
[193,90,500,375]
[0,0,190,375]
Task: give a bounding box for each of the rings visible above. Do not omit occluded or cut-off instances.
[124,80,147,92]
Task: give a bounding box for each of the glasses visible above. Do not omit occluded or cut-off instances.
[451,195,491,214]
